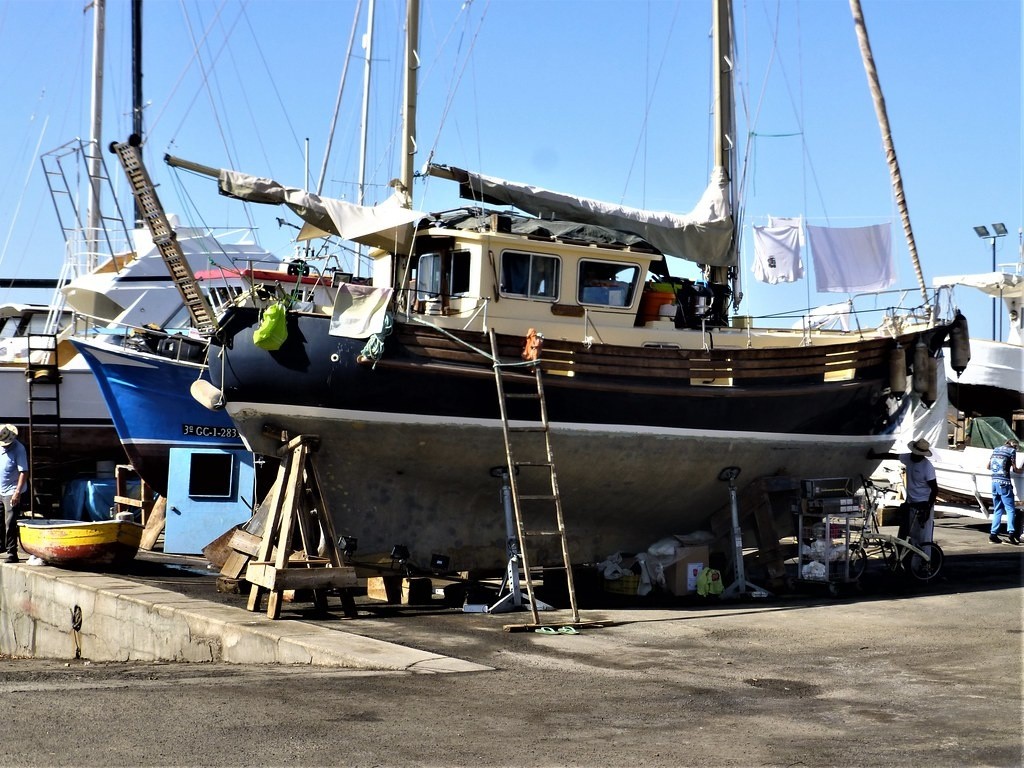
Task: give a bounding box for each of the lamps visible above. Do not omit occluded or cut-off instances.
[338,536,359,565]
[429,553,451,579]
[389,544,415,577]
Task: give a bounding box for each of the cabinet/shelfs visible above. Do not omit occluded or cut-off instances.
[794,513,864,598]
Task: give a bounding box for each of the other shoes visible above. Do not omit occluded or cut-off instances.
[988,534,1002,543]
[5,556,20,563]
[1001,535,1020,545]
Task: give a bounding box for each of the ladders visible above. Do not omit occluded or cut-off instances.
[489,326,614,633]
[24,331,64,521]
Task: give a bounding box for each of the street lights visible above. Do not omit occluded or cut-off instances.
[974,222,1010,341]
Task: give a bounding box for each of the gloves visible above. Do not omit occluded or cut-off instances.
[922,504,931,523]
[865,448,876,460]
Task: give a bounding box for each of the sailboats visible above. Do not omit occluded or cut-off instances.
[0,0,1023,580]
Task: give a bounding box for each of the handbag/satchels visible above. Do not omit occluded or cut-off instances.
[253,294,288,351]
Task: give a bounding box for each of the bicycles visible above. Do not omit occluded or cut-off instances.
[829,483,945,582]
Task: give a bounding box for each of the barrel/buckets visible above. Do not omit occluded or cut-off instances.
[96,461,115,479]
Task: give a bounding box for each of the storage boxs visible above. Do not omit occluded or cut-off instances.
[606,574,641,596]
[664,545,711,596]
[814,496,860,513]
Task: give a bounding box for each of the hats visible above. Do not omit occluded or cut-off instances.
[907,438,933,457]
[0,423,18,447]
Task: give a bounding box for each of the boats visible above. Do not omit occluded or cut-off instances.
[16,515,146,571]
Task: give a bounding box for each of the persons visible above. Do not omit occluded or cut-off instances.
[867,438,939,569]
[986,439,1024,545]
[0,424,28,563]
